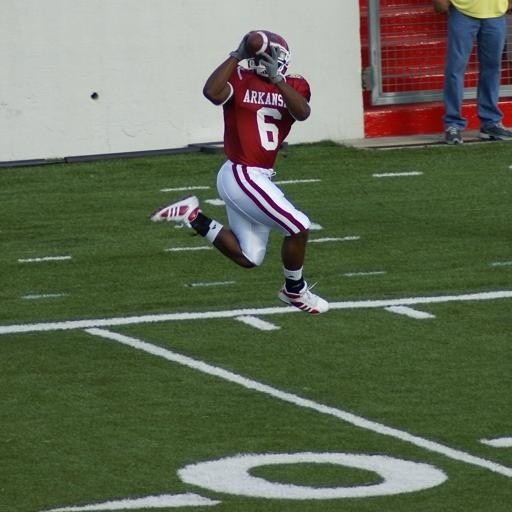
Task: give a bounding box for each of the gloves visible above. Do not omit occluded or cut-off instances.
[259,46,283,83]
[229,30,253,62]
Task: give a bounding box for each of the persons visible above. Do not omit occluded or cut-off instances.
[434,0,511,149]
[151,29,334,314]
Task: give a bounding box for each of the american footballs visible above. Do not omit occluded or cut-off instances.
[246,31,281,61]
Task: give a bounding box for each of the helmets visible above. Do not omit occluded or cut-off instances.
[248,30,291,78]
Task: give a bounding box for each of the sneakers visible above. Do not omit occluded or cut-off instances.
[278,280,329,316]
[478,124,512,141]
[148,194,203,229]
[444,127,465,145]
